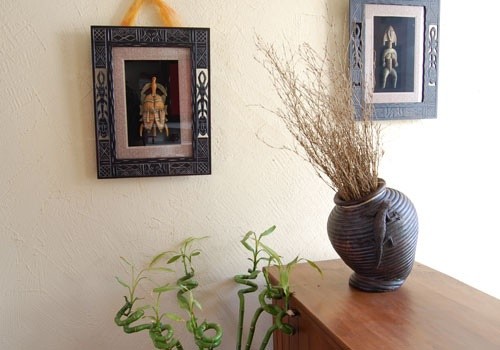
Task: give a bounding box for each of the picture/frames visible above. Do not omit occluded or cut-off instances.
[91,25,212,179]
[348,0,440,120]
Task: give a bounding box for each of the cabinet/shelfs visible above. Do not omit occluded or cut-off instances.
[262,257,500,349]
